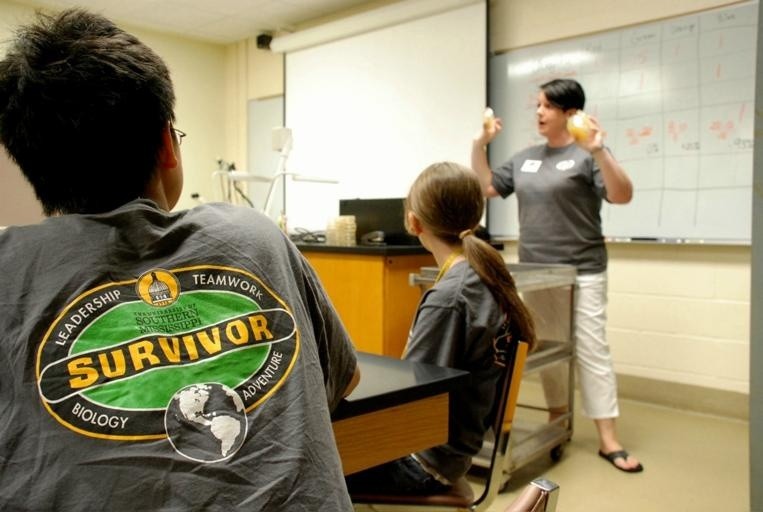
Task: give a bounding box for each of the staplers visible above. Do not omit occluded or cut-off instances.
[361,230,388,246]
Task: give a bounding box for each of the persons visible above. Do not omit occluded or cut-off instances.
[1,8,362,512]
[341,162,542,494]
[472,78,645,474]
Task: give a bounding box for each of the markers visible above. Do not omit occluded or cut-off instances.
[631,237,659,241]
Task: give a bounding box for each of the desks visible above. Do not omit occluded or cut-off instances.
[332,350,470,476]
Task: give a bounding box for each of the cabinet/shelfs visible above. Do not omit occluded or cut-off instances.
[409,264,577,494]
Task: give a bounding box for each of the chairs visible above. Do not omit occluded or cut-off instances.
[350,337,529,512]
[503,477,560,512]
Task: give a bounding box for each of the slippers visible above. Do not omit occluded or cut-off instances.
[599,447,643,472]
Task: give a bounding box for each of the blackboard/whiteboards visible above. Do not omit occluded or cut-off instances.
[245,0,760,247]
